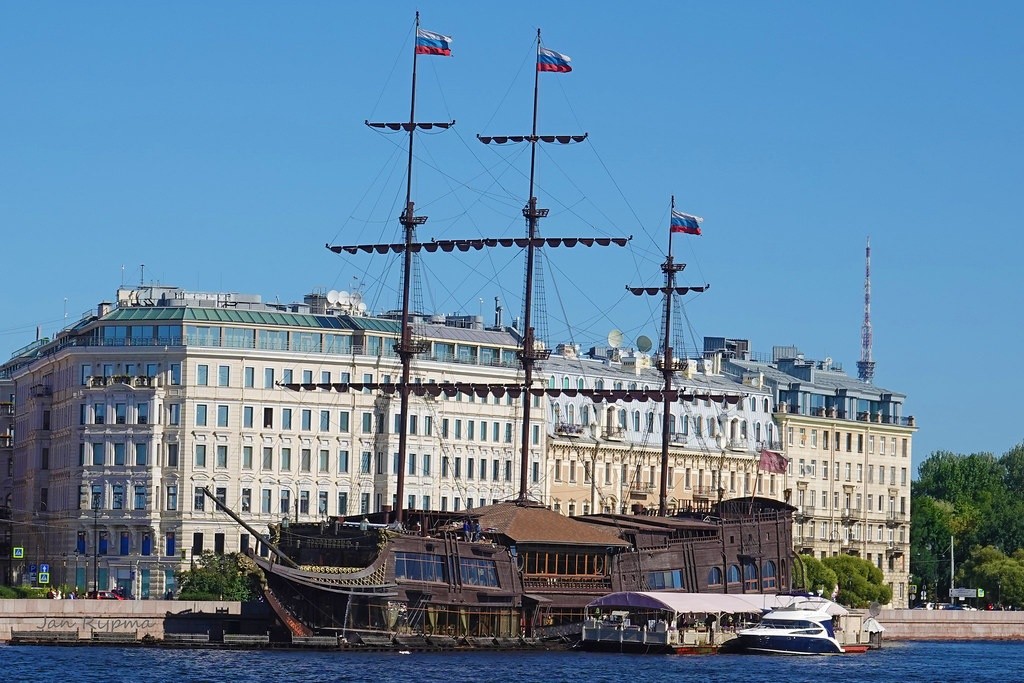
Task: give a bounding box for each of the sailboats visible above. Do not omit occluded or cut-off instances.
[200,7,888,663]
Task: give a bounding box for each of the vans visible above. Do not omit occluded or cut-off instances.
[90,590,124,600]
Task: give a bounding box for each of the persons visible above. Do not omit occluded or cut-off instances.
[546,616,554,626]
[983,600,1022,611]
[391,518,486,542]
[43,584,181,601]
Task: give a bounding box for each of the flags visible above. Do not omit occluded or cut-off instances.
[758,448,790,475]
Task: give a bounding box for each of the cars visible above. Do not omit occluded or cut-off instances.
[912,602,1004,610]
